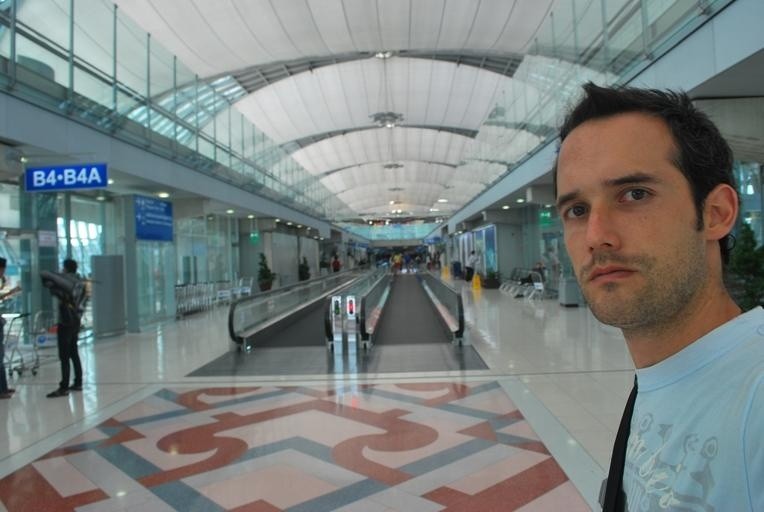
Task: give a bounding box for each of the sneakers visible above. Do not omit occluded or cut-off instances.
[46,385,83,399]
[0,387,16,400]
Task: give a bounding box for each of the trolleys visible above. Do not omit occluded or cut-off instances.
[176,277,253,318]
[4,308,90,376]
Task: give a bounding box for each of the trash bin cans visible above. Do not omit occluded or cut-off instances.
[450,261,461,279]
[558,278,580,307]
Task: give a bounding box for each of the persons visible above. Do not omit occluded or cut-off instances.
[533,260,547,289]
[332,255,340,274]
[385,251,441,274]
[555,83,764,512]
[0,258,88,398]
[464,251,478,282]
